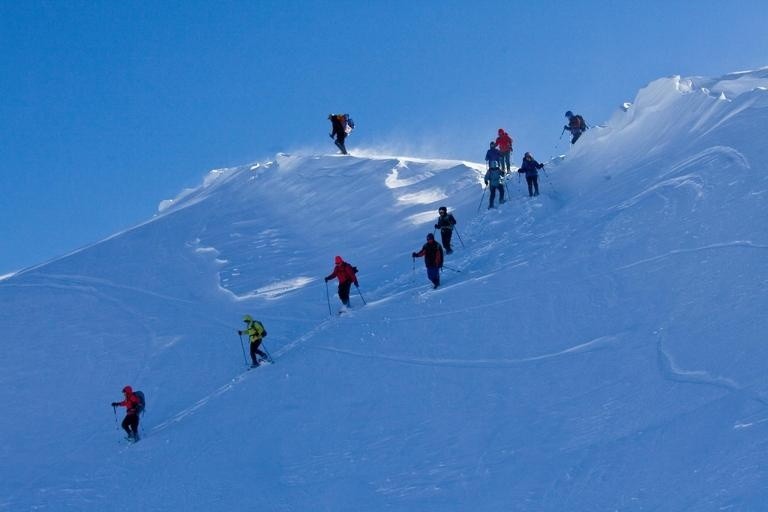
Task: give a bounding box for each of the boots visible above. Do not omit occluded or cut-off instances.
[502,168,511,174]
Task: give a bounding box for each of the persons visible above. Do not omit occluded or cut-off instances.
[238,316,271,369]
[324,255,359,309]
[483,160,508,211]
[482,141,504,167]
[327,114,349,156]
[412,233,445,292]
[432,206,456,255]
[110,383,143,442]
[494,129,514,176]
[562,110,586,145]
[518,153,545,197]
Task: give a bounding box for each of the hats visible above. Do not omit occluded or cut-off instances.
[491,160,499,168]
[335,255,342,264]
[427,233,434,240]
[524,152,531,159]
[565,110,573,118]
[327,113,334,119]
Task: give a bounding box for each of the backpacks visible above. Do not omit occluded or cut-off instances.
[252,320,267,337]
[338,113,353,133]
[132,391,145,412]
[576,115,586,130]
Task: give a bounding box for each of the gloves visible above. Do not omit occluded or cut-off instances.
[325,277,328,282]
[355,281,359,287]
[435,224,438,228]
[239,331,242,335]
[112,403,117,406]
[412,252,416,257]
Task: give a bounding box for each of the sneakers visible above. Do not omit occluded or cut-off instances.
[129,432,138,441]
[250,362,259,368]
